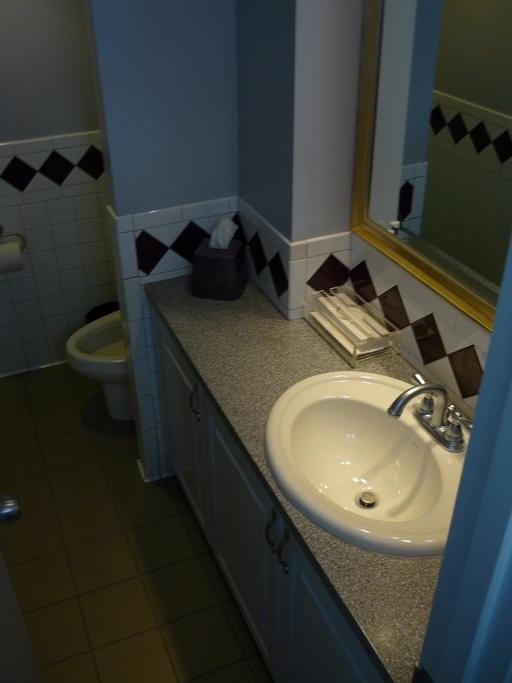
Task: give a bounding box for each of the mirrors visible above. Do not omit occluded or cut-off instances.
[351,0,512,333]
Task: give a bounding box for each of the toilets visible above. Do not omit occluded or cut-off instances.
[64,309,135,420]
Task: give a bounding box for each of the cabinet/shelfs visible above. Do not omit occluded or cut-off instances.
[150,301,210,546]
[202,383,393,683]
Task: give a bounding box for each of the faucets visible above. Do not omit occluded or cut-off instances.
[386,373,474,453]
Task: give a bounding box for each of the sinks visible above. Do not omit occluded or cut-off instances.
[264,370,472,557]
